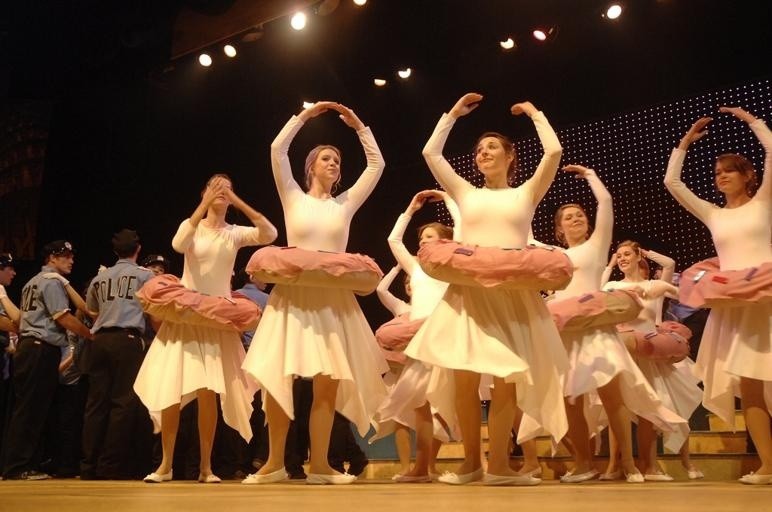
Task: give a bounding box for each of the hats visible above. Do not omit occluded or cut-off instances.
[0,253,13,264]
[141,255,168,274]
[44,240,72,256]
[112,229,139,250]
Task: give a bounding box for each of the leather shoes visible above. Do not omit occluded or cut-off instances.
[348,458,368,476]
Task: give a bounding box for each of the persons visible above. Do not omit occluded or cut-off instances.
[421,91,565,486]
[541,164,644,483]
[377,189,703,483]
[2,231,370,481]
[242,101,388,483]
[133,175,277,483]
[662,104,771,484]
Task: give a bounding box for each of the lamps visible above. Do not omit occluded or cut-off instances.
[600,1,626,25]
[496,17,559,53]
[371,55,413,87]
[175,0,368,68]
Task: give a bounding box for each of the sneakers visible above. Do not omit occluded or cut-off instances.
[199,472,221,482]
[241,466,288,484]
[8,464,48,479]
[143,468,173,482]
[306,472,357,484]
[738,471,772,484]
